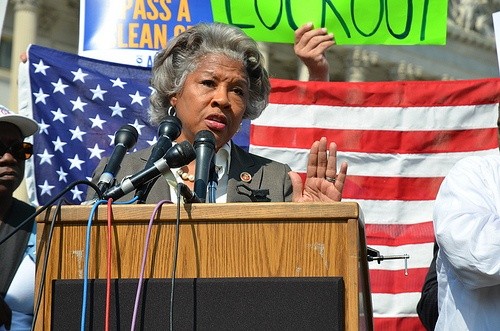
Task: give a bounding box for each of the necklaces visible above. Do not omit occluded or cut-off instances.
[177,169,194,182]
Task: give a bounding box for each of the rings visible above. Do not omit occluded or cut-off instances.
[326,176,335,182]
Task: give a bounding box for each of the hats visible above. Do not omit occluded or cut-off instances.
[0,104,38,138]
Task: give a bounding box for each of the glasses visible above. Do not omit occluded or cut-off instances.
[0,139,34,161]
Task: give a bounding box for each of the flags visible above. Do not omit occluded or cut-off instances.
[19,43,500,331]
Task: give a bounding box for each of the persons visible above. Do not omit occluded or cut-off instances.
[0,104,37,331]
[433,99,500,331]
[87,19,348,205]
[293,22,336,81]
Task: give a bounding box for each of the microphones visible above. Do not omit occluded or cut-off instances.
[134,115,183,204]
[193,129,216,202]
[93,125,138,201]
[102,139,197,202]
